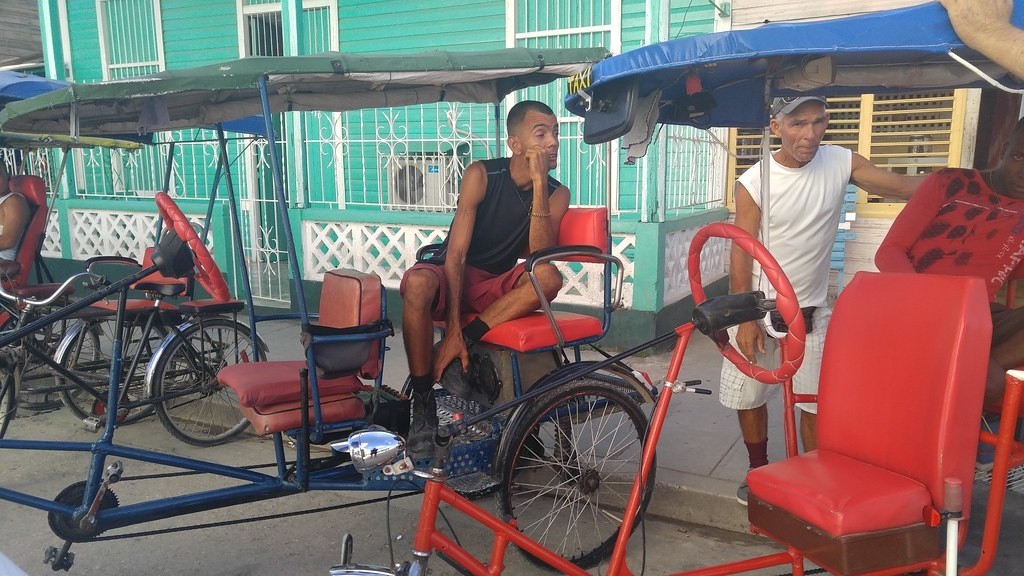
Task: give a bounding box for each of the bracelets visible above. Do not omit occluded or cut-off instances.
[530,212,552,217]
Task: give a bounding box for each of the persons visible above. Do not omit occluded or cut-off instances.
[873,117,1024,386]
[400,100,569,459]
[0,159,28,262]
[720,94,928,507]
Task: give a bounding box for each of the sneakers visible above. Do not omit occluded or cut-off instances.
[736,468,755,507]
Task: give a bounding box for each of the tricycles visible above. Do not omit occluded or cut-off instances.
[1,68,273,445]
[0,49,659,576]
[324,0,1020,576]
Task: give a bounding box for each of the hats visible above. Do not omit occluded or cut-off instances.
[773,94,829,116]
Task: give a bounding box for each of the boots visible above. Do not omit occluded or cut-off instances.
[406,389,438,459]
[431,332,477,399]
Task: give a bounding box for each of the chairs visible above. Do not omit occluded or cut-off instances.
[85,247,193,321]
[239,269,381,436]
[746,271,993,575]
[2,175,48,294]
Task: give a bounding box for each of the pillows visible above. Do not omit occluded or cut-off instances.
[217,361,371,407]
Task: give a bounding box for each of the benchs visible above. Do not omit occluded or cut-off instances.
[416,207,607,351]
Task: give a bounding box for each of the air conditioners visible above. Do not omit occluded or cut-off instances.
[388,160,456,211]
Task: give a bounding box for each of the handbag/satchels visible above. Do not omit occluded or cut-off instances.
[763,306,816,339]
